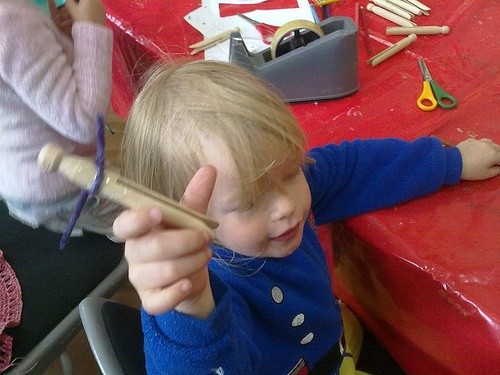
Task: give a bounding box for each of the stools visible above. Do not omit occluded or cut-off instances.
[0,201,124,371]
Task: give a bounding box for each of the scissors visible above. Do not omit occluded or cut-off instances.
[417,59,458,112]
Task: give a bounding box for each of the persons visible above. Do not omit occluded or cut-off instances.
[0,1,133,235]
[112,59,500,374]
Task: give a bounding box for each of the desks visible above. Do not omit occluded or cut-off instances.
[82,1,500,375]
